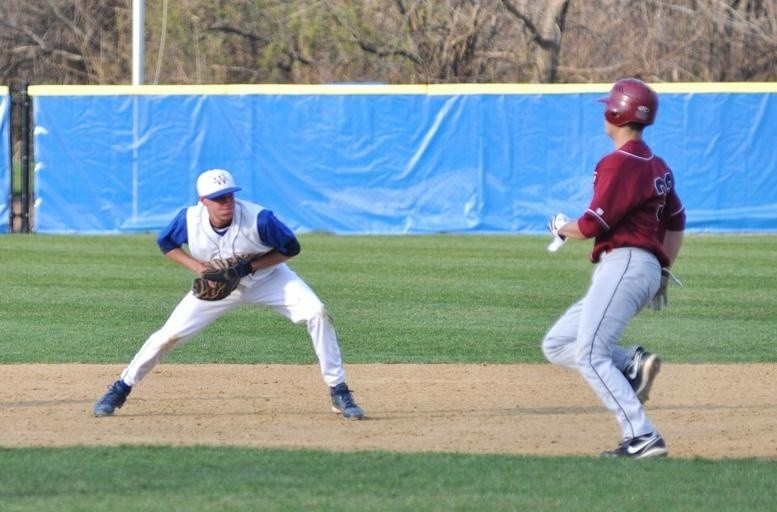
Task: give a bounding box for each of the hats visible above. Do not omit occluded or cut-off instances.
[197,169,242,199]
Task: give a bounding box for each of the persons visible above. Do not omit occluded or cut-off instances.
[91,168,364,421]
[540,77,687,458]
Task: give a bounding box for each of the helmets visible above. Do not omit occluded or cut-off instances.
[596,78,657,126]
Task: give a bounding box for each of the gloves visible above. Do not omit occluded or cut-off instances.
[546,214,571,242]
[646,268,671,311]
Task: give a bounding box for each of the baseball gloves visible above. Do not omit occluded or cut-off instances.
[192,257,251,300]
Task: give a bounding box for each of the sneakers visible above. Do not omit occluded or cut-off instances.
[600,431,668,460]
[93,382,127,415]
[623,350,660,405]
[331,384,364,418]
